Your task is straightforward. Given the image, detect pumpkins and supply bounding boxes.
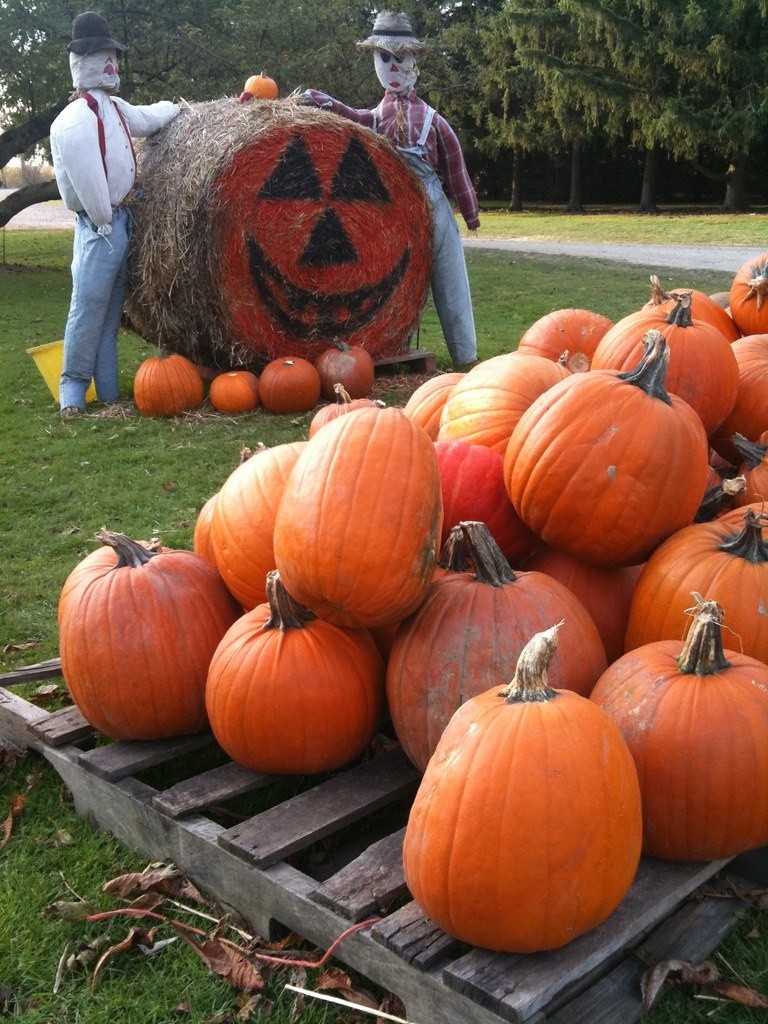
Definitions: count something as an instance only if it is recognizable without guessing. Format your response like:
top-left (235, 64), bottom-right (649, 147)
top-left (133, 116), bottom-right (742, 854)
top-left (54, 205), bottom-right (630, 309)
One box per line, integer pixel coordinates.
top-left (58, 252), bottom-right (768, 951)
top-left (244, 70), bottom-right (278, 99)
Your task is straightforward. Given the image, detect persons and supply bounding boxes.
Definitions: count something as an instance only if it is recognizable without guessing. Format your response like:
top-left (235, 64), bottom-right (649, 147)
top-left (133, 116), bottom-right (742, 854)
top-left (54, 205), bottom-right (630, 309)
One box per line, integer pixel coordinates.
top-left (301, 8), bottom-right (482, 372)
top-left (49, 12), bottom-right (181, 419)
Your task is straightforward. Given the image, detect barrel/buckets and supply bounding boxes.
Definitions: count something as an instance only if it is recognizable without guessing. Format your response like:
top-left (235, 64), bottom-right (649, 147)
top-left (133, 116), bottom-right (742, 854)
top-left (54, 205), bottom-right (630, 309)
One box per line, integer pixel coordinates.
top-left (25, 340), bottom-right (97, 405)
